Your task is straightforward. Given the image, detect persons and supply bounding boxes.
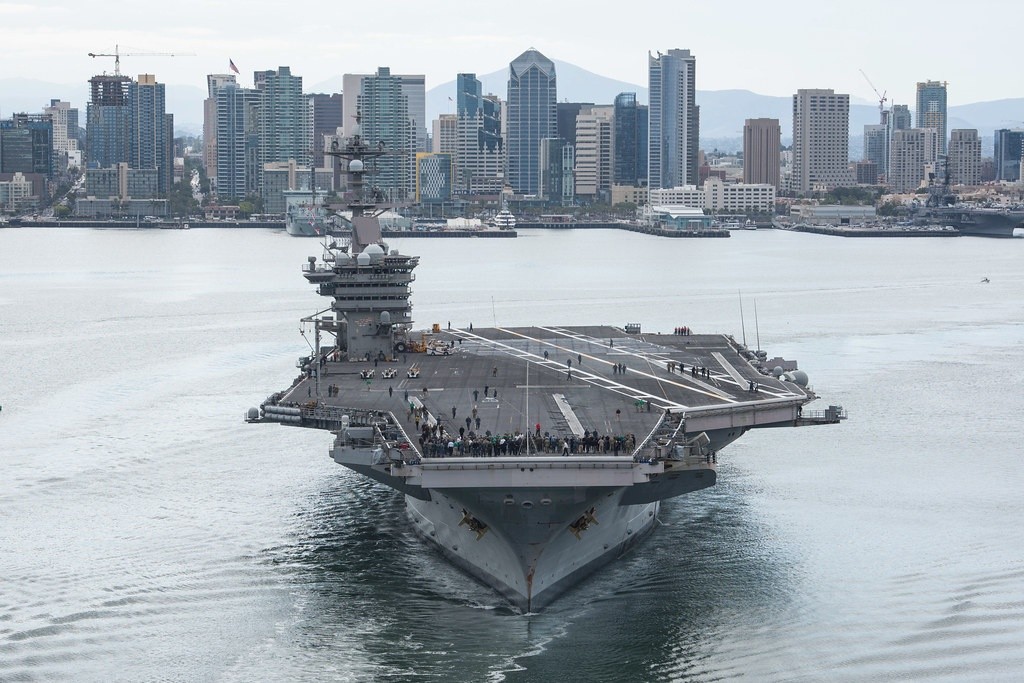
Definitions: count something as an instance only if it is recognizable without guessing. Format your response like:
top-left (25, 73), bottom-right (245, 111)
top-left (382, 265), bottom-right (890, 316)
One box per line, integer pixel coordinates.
top-left (307, 321), bottom-right (650, 457)
top-left (665, 326), bottom-right (761, 392)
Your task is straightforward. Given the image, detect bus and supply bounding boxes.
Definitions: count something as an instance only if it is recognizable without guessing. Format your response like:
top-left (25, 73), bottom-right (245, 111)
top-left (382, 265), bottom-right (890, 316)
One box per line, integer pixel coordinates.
top-left (250, 213), bottom-right (283, 223)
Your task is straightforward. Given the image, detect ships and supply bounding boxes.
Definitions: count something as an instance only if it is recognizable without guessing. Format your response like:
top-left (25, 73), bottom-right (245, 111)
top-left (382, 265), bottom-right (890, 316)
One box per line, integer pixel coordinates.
top-left (242, 133), bottom-right (849, 615)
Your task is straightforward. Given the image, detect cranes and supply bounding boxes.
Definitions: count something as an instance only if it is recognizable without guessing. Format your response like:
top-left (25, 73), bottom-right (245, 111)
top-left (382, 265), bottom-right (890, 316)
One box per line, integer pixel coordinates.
top-left (88, 43), bottom-right (175, 77)
top-left (858, 68), bottom-right (888, 125)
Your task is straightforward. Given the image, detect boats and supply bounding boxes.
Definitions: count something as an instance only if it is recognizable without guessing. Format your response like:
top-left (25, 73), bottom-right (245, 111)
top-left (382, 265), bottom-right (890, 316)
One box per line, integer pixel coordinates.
top-left (282, 186), bottom-right (332, 237)
top-left (493, 197), bottom-right (517, 231)
top-left (708, 215), bottom-right (741, 230)
top-left (743, 217), bottom-right (757, 230)
